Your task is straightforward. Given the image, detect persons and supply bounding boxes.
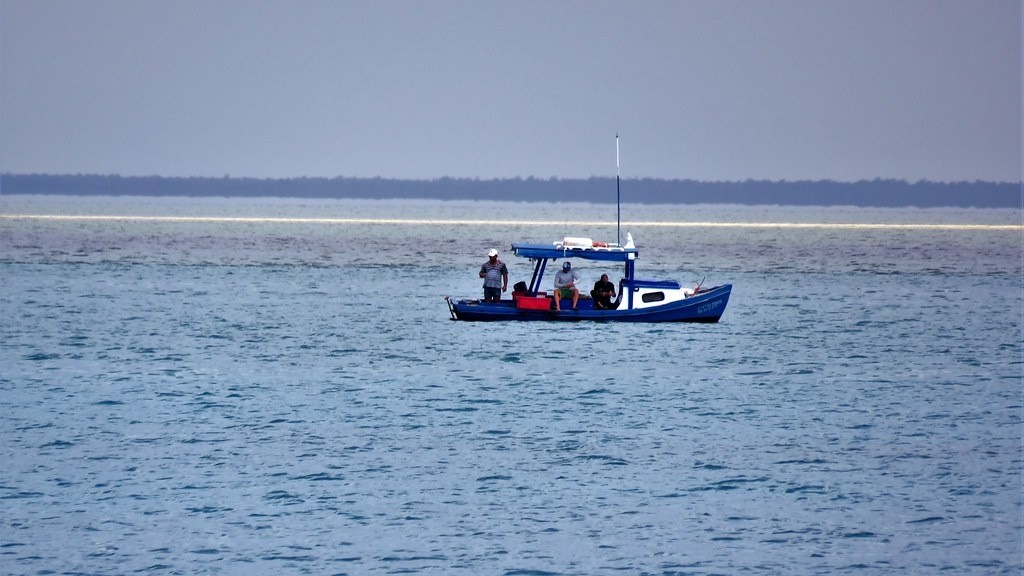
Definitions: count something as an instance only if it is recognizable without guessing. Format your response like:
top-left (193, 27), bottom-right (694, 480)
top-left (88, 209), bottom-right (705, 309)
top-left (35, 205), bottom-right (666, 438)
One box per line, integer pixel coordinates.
top-left (553, 261), bottom-right (582, 311)
top-left (590, 274), bottom-right (616, 310)
top-left (479, 249), bottom-right (508, 304)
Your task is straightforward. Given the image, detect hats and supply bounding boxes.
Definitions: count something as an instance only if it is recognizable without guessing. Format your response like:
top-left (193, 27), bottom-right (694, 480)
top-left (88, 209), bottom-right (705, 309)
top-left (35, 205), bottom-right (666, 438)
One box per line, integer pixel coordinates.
top-left (563, 262), bottom-right (571, 270)
top-left (488, 249), bottom-right (498, 257)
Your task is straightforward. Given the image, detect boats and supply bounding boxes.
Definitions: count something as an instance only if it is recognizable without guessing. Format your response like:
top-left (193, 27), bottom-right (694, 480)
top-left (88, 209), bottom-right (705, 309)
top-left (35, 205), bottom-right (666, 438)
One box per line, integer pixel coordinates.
top-left (445, 132), bottom-right (732, 323)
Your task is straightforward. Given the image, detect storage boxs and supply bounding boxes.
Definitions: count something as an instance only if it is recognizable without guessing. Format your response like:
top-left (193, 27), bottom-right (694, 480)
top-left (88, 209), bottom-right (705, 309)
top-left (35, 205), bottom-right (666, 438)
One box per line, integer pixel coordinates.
top-left (512, 291), bottom-right (547, 300)
top-left (516, 295), bottom-right (551, 310)
